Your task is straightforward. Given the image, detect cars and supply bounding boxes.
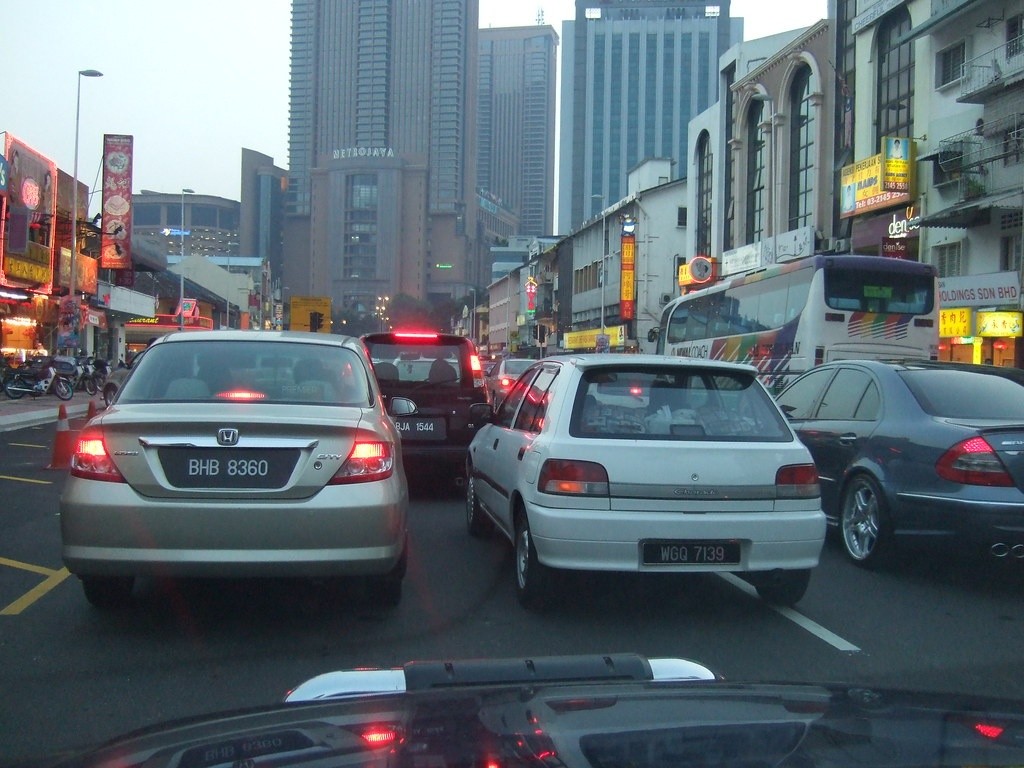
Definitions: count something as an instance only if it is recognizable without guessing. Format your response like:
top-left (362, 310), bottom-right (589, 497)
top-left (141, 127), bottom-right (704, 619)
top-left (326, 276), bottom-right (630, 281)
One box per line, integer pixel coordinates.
top-left (772, 357), bottom-right (1023, 573)
top-left (102, 349), bottom-right (146, 408)
top-left (586, 381), bottom-right (652, 410)
top-left (480, 358), bottom-right (538, 413)
top-left (60, 330), bottom-right (410, 610)
top-left (465, 354), bottom-right (828, 616)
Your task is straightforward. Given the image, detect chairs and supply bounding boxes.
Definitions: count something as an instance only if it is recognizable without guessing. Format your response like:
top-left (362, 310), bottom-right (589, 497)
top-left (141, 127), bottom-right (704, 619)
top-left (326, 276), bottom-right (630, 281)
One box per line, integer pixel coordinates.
top-left (164, 379), bottom-right (213, 400)
top-left (374, 362), bottom-right (399, 387)
top-left (682, 298), bottom-right (925, 341)
top-left (294, 381), bottom-right (338, 403)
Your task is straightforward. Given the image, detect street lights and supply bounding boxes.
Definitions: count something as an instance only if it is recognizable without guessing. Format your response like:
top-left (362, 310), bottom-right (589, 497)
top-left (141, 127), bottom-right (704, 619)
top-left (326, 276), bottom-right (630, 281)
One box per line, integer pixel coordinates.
top-left (469, 289), bottom-right (476, 339)
top-left (225, 242), bottom-right (240, 330)
top-left (259, 269), bottom-right (268, 330)
top-left (751, 94), bottom-right (778, 265)
top-left (374, 296), bottom-right (390, 332)
top-left (179, 188), bottom-right (196, 330)
top-left (68, 69), bottom-right (105, 357)
top-left (590, 195), bottom-right (606, 334)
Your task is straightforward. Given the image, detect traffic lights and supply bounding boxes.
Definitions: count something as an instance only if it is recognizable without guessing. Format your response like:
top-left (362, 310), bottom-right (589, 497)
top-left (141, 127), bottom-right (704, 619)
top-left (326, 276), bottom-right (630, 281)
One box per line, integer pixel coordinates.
top-left (315, 312), bottom-right (324, 329)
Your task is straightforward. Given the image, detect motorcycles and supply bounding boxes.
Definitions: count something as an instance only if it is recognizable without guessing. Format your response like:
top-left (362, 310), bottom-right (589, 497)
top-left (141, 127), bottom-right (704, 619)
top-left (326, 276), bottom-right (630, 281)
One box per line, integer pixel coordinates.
top-left (0, 350), bottom-right (130, 401)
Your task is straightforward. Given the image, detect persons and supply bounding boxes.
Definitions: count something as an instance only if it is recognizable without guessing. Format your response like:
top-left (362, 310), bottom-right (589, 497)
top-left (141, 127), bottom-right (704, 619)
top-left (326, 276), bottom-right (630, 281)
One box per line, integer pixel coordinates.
top-left (36, 343), bottom-right (47, 356)
top-left (858, 176), bottom-right (877, 189)
top-left (844, 186), bottom-right (852, 212)
top-left (646, 380), bottom-right (689, 417)
top-left (199, 362), bottom-right (224, 396)
top-left (293, 359), bottom-right (322, 385)
top-left (890, 140), bottom-right (903, 159)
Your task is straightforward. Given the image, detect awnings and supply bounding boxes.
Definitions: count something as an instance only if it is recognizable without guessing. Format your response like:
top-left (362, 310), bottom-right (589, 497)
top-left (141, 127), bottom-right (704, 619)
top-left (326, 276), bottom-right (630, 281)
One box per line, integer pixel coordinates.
top-left (915, 141), bottom-right (963, 161)
top-left (907, 190), bottom-right (1024, 229)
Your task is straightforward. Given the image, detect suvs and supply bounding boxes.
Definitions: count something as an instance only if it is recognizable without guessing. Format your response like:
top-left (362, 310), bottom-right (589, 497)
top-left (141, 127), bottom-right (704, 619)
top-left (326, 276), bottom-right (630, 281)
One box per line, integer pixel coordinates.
top-left (359, 332), bottom-right (495, 498)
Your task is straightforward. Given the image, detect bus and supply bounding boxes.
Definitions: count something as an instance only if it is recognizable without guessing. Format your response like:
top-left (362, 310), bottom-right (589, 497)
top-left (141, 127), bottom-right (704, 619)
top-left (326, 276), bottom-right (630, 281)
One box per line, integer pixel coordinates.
top-left (647, 254), bottom-right (941, 417)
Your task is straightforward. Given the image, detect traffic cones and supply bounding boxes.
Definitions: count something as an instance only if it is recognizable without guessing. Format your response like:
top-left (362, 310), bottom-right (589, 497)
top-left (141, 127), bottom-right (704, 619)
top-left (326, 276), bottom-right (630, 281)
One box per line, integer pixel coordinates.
top-left (86, 401), bottom-right (98, 422)
top-left (44, 404), bottom-right (74, 470)
